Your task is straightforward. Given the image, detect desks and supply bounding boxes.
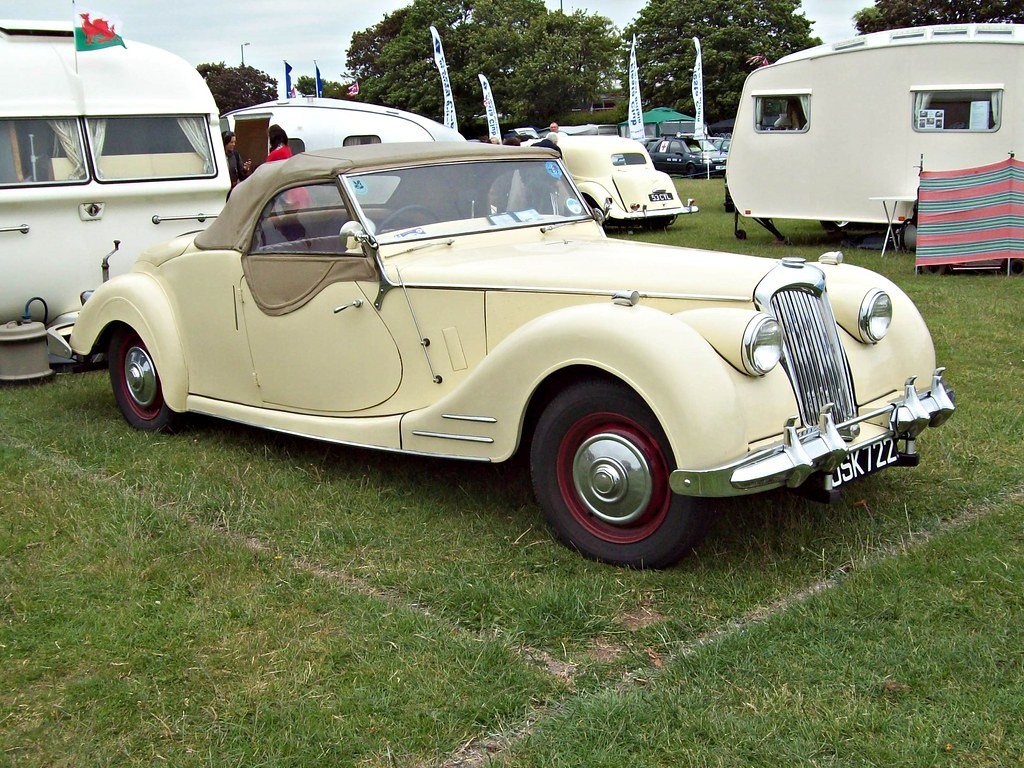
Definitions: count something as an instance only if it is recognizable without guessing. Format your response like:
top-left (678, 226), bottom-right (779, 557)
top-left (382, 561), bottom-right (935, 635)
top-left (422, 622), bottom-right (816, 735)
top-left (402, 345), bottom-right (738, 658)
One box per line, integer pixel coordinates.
top-left (869, 197), bottom-right (918, 257)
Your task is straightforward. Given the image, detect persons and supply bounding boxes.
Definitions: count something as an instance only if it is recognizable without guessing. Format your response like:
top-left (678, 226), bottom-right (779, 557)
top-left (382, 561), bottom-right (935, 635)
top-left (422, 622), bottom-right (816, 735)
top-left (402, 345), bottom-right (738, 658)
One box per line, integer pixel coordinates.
top-left (263, 124), bottom-right (292, 211)
top-left (222, 131), bottom-right (252, 187)
top-left (479, 136), bottom-right (493, 144)
top-left (519, 132), bottom-right (562, 214)
top-left (550, 122), bottom-right (569, 136)
top-left (489, 137), bottom-right (499, 144)
top-left (502, 136), bottom-right (520, 146)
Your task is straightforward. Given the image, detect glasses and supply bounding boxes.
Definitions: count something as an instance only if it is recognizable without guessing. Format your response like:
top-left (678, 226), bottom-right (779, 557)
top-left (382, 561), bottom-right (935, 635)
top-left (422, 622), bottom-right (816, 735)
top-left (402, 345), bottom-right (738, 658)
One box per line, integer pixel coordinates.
top-left (222, 131), bottom-right (233, 141)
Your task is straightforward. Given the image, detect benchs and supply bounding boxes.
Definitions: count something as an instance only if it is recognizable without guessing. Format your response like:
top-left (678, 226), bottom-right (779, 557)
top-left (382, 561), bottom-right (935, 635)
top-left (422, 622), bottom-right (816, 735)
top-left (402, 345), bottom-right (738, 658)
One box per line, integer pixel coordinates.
top-left (258, 235), bottom-right (346, 254)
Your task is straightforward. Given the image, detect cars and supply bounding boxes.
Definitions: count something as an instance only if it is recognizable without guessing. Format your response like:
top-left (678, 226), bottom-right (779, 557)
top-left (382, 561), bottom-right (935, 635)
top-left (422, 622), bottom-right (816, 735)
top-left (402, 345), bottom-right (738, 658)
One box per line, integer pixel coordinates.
top-left (503, 126), bottom-right (540, 143)
top-left (46, 141), bottom-right (956, 567)
top-left (521, 136), bottom-right (698, 227)
top-left (639, 135), bottom-right (731, 178)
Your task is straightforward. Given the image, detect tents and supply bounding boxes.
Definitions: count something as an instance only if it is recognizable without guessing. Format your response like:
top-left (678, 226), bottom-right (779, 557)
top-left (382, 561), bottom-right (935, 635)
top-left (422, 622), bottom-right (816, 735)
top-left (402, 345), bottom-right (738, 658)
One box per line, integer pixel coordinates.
top-left (618, 107), bottom-right (707, 138)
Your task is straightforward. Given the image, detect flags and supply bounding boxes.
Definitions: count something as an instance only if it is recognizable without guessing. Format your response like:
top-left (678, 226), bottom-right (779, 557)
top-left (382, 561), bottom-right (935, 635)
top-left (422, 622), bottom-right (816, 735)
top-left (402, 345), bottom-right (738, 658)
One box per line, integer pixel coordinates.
top-left (72, 2), bottom-right (127, 51)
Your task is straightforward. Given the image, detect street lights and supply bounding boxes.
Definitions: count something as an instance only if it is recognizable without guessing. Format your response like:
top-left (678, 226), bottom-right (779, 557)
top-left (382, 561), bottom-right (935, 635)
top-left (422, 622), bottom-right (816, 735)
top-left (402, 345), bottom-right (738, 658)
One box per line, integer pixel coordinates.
top-left (240, 43), bottom-right (252, 64)
